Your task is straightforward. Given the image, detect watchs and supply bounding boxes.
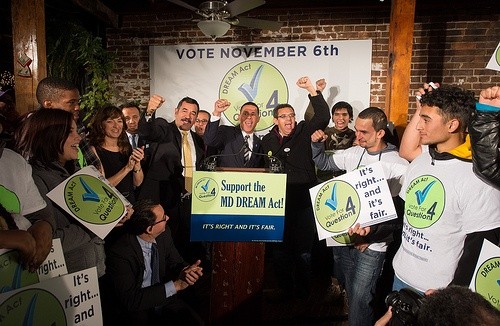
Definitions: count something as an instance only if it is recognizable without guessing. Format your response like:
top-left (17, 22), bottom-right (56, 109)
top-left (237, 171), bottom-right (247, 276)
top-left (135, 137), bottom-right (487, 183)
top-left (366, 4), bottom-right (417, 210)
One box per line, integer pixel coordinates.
top-left (132, 167), bottom-right (142, 174)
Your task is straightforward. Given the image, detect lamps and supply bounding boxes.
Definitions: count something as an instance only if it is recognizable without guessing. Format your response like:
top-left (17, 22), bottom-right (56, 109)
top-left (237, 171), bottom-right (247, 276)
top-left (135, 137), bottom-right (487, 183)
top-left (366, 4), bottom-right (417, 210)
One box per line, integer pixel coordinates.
top-left (197, 17), bottom-right (231, 42)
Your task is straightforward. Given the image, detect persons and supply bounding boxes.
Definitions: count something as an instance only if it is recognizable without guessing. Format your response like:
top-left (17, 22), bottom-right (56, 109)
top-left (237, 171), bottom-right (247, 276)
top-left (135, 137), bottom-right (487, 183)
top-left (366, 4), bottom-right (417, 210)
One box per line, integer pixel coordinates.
top-left (0, 76), bottom-right (500, 326)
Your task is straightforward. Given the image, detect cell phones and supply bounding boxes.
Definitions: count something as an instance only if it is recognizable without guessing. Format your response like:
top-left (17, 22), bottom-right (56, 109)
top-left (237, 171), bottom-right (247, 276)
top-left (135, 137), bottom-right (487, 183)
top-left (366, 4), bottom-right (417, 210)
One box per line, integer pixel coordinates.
top-left (415, 82), bottom-right (438, 101)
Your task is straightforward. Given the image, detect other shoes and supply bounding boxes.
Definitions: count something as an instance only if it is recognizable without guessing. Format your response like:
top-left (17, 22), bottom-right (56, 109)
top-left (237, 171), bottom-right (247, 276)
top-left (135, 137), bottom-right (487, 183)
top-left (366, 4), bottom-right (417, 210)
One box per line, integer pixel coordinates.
top-left (263, 287), bottom-right (287, 299)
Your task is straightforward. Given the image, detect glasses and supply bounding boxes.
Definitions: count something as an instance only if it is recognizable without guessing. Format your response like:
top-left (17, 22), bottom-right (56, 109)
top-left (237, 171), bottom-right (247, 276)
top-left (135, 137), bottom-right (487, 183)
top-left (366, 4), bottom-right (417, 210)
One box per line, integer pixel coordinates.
top-left (152, 215), bottom-right (167, 226)
top-left (194, 119), bottom-right (208, 125)
top-left (277, 114), bottom-right (296, 118)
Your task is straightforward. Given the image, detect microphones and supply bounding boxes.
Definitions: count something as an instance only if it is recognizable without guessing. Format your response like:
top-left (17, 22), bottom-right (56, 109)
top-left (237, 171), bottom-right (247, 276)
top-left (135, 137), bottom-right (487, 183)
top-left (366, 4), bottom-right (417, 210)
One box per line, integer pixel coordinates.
top-left (203, 142), bottom-right (282, 167)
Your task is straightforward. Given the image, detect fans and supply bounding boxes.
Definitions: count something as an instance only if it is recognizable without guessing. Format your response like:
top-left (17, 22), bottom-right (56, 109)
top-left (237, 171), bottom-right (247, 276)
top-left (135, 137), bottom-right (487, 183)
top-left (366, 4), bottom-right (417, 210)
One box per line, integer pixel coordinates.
top-left (167, 0), bottom-right (284, 32)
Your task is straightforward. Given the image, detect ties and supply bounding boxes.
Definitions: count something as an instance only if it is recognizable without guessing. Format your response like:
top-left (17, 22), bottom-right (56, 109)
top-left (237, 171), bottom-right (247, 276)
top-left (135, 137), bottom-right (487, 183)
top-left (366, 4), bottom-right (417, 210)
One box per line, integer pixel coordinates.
top-left (148, 243), bottom-right (160, 286)
top-left (243, 135), bottom-right (251, 166)
top-left (181, 131), bottom-right (193, 194)
top-left (131, 134), bottom-right (137, 149)
